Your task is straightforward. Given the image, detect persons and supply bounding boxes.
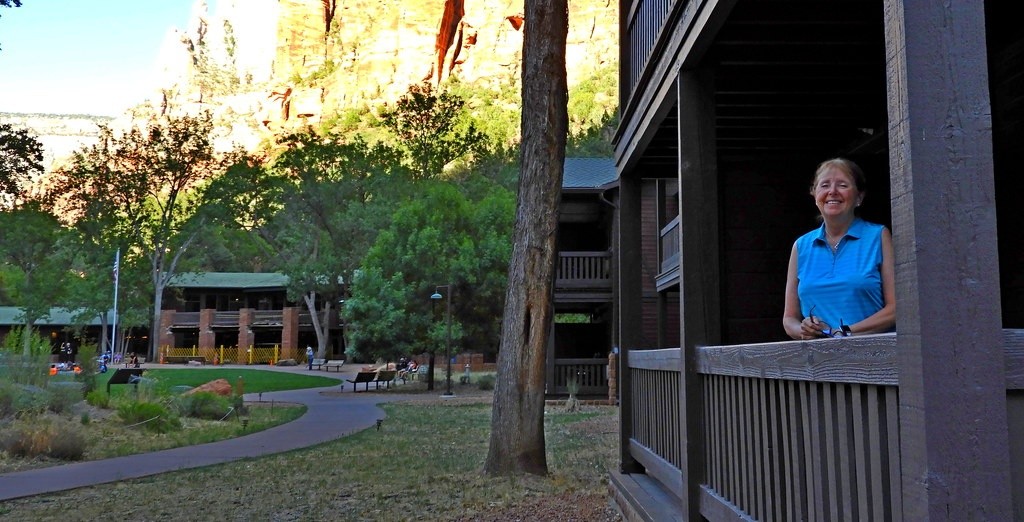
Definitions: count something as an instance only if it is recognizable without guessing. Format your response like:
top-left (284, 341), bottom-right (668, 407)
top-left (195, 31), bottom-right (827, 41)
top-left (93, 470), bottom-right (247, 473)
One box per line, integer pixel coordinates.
top-left (61, 342), bottom-right (140, 377)
top-left (782, 157), bottom-right (896, 341)
top-left (306, 347), bottom-right (313, 370)
top-left (396, 357), bottom-right (417, 379)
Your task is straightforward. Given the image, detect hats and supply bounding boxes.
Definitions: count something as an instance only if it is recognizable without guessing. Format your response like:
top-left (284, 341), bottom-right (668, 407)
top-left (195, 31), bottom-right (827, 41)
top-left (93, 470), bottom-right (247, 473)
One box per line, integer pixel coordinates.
top-left (400, 358), bottom-right (404, 360)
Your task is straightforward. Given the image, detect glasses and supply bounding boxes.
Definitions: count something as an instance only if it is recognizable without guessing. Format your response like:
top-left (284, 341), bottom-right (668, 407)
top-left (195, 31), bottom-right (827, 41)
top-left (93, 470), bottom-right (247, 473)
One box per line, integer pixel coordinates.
top-left (809, 304), bottom-right (846, 337)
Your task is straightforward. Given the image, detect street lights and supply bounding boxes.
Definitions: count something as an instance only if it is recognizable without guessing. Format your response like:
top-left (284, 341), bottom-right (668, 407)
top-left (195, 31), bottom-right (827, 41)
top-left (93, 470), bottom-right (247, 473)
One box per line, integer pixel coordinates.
top-left (431, 285), bottom-right (451, 395)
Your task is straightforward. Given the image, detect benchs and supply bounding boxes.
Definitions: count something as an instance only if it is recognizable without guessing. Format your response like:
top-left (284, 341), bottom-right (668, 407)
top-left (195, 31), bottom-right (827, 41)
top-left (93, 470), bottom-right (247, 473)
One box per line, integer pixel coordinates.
top-left (123, 362), bottom-right (144, 368)
top-left (219, 360), bottom-right (232, 365)
top-left (306, 359), bottom-right (326, 370)
top-left (166, 356), bottom-right (209, 366)
top-left (346, 372), bottom-right (377, 393)
top-left (373, 371), bottom-right (397, 390)
top-left (323, 360), bottom-right (345, 372)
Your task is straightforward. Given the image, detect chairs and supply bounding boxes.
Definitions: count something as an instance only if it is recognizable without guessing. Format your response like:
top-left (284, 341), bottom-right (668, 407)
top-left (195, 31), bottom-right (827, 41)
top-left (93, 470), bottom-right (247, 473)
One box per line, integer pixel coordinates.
top-left (113, 352), bottom-right (123, 365)
